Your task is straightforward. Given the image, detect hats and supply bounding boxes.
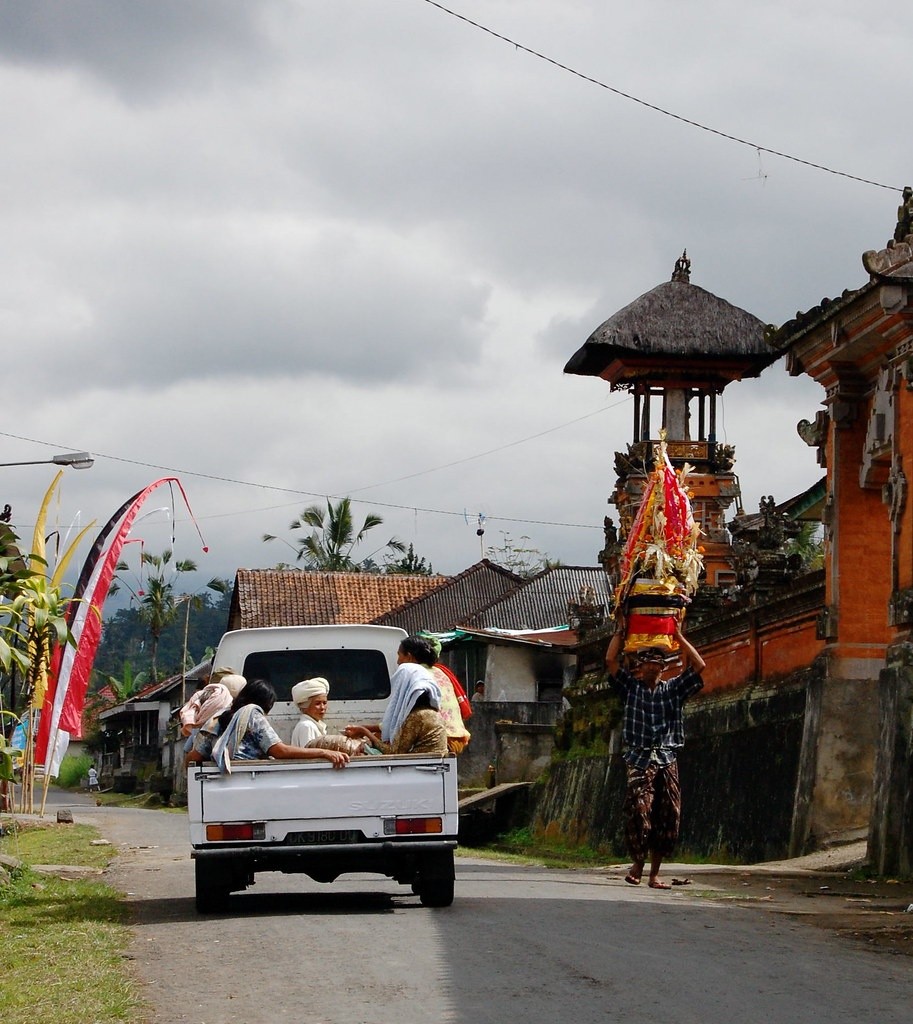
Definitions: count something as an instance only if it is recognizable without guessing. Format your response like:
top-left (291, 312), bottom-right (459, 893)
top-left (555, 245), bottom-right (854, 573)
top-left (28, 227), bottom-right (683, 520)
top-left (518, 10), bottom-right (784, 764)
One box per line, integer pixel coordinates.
top-left (476, 680), bottom-right (484, 686)
top-left (416, 632), bottom-right (442, 657)
top-left (637, 649), bottom-right (664, 667)
top-left (210, 667), bottom-right (240, 683)
top-left (220, 675), bottom-right (247, 698)
top-left (292, 677), bottom-right (330, 710)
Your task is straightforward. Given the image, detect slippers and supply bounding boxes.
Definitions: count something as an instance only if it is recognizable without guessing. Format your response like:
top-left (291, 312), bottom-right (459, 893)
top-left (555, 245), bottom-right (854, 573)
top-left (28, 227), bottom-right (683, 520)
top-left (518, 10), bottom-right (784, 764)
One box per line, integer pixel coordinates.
top-left (625, 870), bottom-right (642, 885)
top-left (648, 879), bottom-right (672, 889)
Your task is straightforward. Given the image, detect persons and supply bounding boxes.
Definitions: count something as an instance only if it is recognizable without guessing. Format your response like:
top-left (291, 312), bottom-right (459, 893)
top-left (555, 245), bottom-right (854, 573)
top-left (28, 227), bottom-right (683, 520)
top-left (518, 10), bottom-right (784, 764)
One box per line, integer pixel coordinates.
top-left (180, 667), bottom-right (247, 767)
top-left (213, 679), bottom-right (350, 775)
top-left (471, 681), bottom-right (485, 700)
top-left (396, 635), bottom-right (471, 754)
top-left (344, 663), bottom-right (448, 754)
top-left (88, 765), bottom-right (101, 793)
top-left (605, 608), bottom-right (706, 891)
top-left (418, 632), bottom-right (472, 720)
top-left (291, 678), bottom-right (329, 747)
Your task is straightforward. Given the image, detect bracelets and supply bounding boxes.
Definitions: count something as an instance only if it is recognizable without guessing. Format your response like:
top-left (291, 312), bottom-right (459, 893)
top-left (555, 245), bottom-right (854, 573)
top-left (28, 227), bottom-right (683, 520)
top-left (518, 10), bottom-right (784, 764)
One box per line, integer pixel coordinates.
top-left (614, 630), bottom-right (623, 636)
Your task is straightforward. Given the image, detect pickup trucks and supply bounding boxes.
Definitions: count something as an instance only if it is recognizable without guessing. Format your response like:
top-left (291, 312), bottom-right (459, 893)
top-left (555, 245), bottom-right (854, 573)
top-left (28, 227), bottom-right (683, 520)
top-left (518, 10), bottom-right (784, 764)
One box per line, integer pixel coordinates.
top-left (188, 623), bottom-right (461, 918)
